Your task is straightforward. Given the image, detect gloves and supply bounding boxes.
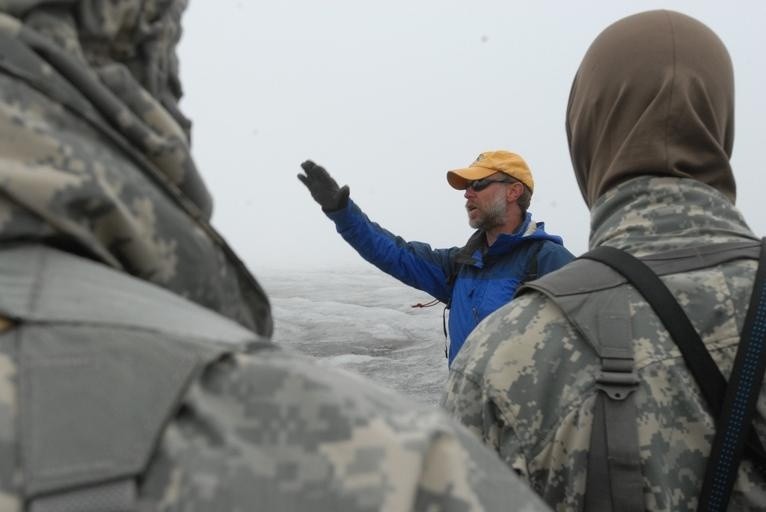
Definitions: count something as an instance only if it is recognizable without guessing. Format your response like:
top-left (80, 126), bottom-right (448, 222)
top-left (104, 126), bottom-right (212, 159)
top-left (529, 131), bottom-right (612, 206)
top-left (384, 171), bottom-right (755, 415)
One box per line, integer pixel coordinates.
top-left (297, 159), bottom-right (350, 212)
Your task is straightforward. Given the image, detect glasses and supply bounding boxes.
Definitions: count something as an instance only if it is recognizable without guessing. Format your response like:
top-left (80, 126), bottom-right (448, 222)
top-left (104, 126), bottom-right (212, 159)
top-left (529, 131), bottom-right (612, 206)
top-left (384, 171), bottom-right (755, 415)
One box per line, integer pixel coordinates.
top-left (464, 178), bottom-right (514, 192)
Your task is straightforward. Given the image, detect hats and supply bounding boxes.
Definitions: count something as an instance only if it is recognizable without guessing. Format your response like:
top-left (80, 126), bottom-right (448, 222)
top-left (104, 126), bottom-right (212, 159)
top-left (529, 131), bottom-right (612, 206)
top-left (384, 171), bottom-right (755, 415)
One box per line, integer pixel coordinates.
top-left (447, 150), bottom-right (535, 192)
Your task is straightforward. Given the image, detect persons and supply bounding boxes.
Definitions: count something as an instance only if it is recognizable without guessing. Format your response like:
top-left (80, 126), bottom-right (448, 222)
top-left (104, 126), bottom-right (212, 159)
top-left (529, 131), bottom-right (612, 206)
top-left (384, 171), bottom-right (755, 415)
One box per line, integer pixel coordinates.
top-left (441, 9), bottom-right (765, 510)
top-left (0, 0), bottom-right (554, 511)
top-left (298, 150), bottom-right (579, 372)
